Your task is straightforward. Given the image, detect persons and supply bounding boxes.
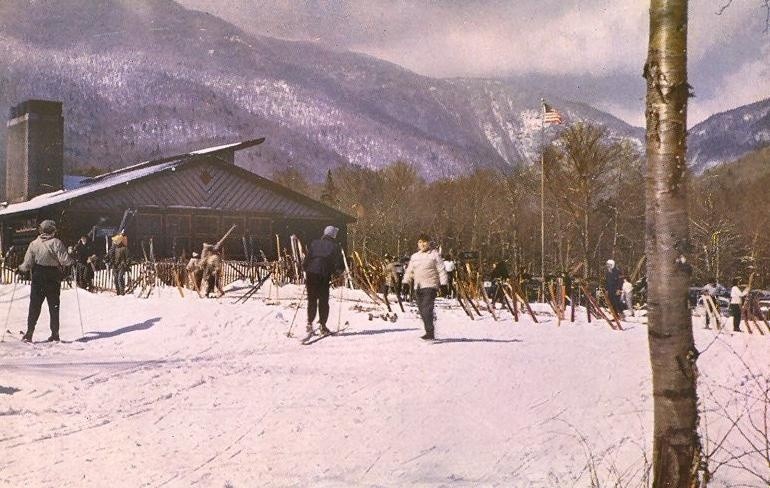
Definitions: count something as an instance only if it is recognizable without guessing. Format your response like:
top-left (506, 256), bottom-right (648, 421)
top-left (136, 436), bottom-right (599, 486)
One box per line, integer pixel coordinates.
top-left (301, 225), bottom-right (344, 334)
top-left (605, 259), bottom-right (626, 321)
top-left (13, 220), bottom-right (76, 343)
top-left (382, 233), bottom-right (456, 340)
top-left (701, 277), bottom-right (726, 330)
top-left (490, 260), bottom-right (509, 309)
top-left (620, 275), bottom-right (635, 317)
top-left (730, 279), bottom-right (750, 331)
top-left (73, 234), bottom-right (96, 291)
top-left (197, 245), bottom-right (225, 298)
top-left (105, 234), bottom-right (131, 296)
top-left (186, 251), bottom-right (199, 290)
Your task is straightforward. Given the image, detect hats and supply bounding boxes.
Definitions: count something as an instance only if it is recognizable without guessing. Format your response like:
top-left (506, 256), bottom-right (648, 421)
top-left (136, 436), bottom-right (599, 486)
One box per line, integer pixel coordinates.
top-left (324, 226), bottom-right (337, 238)
top-left (40, 220), bottom-right (56, 234)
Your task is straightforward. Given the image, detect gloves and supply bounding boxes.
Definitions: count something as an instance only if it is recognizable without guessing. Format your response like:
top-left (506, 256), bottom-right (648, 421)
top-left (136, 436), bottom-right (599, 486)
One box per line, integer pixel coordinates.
top-left (401, 284), bottom-right (410, 295)
top-left (440, 284), bottom-right (449, 297)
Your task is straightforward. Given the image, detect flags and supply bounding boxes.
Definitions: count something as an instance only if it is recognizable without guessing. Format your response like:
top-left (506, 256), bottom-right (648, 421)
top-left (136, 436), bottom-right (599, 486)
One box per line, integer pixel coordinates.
top-left (542, 103), bottom-right (561, 124)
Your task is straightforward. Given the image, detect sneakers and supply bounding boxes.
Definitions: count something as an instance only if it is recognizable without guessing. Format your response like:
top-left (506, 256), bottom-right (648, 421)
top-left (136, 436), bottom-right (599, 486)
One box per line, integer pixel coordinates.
top-left (306, 324), bottom-right (314, 335)
top-left (48, 333), bottom-right (60, 341)
top-left (316, 322), bottom-right (330, 335)
top-left (22, 333), bottom-right (33, 341)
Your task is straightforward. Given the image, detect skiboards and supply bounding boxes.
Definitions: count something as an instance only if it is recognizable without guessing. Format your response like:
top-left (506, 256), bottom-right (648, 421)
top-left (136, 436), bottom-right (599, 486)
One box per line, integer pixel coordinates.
top-left (300, 321), bottom-right (348, 345)
top-left (125, 238), bottom-right (205, 299)
top-left (329, 243), bottom-right (403, 323)
top-left (223, 234), bottom-right (309, 304)
top-left (103, 208), bottom-right (137, 268)
top-left (7, 330), bottom-right (73, 347)
top-left (447, 248), bottom-right (648, 331)
top-left (197, 224), bottom-right (238, 264)
top-left (700, 272), bottom-right (770, 336)
